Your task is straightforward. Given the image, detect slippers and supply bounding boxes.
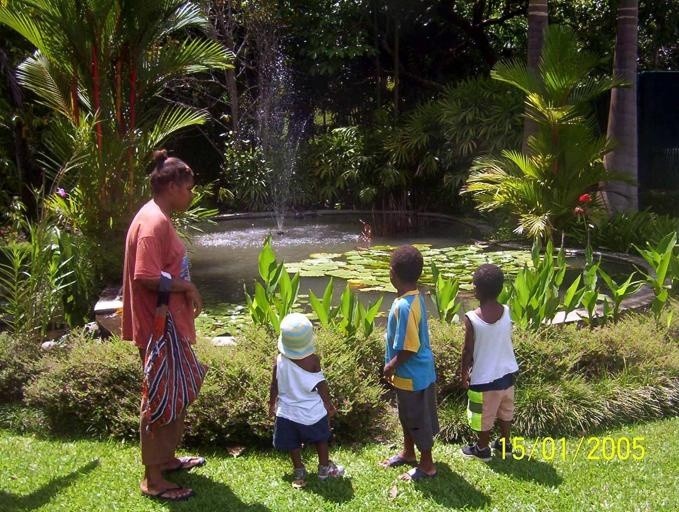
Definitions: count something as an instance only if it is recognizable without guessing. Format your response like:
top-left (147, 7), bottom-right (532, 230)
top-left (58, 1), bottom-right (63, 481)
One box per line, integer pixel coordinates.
top-left (382, 454), bottom-right (416, 467)
top-left (141, 482), bottom-right (195, 501)
top-left (161, 456), bottom-right (205, 472)
top-left (399, 467), bottom-right (437, 482)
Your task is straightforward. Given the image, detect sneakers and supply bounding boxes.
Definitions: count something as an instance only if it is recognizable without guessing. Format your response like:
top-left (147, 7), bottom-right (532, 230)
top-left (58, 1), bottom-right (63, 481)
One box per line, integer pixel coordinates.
top-left (491, 438), bottom-right (512, 456)
top-left (292, 463), bottom-right (308, 488)
top-left (317, 460), bottom-right (344, 481)
top-left (460, 444), bottom-right (492, 462)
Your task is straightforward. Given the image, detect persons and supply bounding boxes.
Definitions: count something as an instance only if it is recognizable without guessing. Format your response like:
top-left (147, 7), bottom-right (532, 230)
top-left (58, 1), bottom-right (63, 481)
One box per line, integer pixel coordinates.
top-left (266, 311), bottom-right (346, 484)
top-left (378, 244), bottom-right (440, 482)
top-left (458, 262), bottom-right (520, 463)
top-left (121, 147), bottom-right (207, 503)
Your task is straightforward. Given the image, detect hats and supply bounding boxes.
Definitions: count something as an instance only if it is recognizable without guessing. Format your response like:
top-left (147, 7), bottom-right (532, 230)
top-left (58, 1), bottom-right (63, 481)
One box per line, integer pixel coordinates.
top-left (278, 313), bottom-right (318, 361)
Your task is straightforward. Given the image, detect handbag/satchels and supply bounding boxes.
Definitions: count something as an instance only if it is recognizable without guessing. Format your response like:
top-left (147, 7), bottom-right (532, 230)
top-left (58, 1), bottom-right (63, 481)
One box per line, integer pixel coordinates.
top-left (145, 311), bottom-right (208, 432)
